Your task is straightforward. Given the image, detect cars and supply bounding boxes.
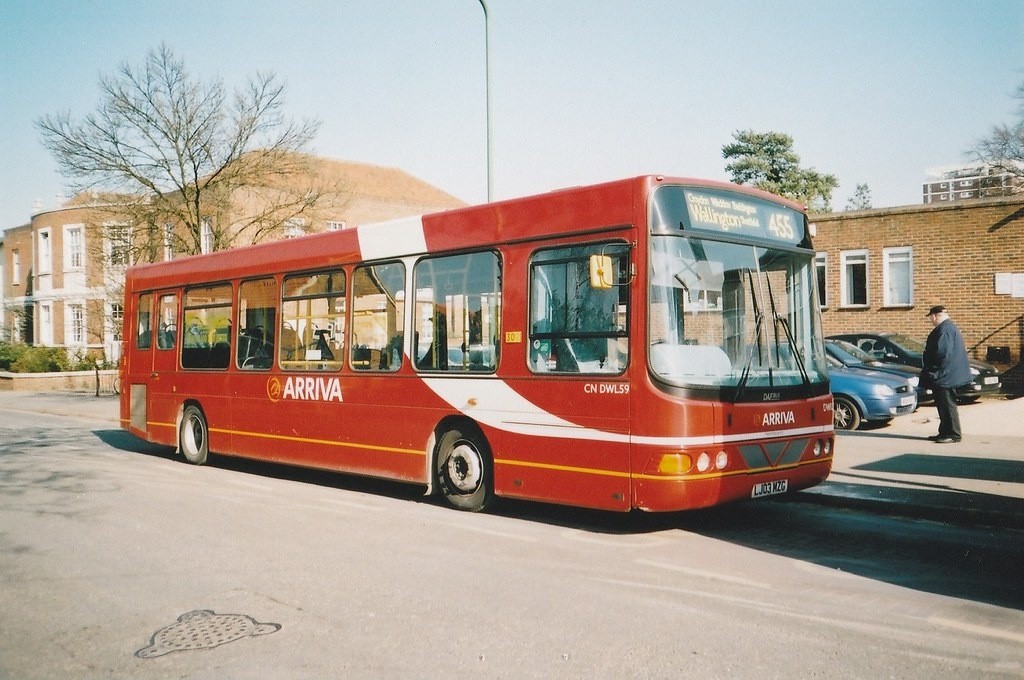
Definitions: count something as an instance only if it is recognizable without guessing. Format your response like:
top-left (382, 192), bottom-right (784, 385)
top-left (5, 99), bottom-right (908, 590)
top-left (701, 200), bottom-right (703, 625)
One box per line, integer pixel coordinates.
top-left (829, 340), bottom-right (934, 403)
top-left (825, 331), bottom-right (1001, 404)
top-left (728, 340), bottom-right (917, 431)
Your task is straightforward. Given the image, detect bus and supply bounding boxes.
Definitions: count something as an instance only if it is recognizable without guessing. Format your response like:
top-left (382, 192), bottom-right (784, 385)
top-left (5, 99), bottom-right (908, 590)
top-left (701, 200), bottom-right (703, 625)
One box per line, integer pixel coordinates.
top-left (119, 174), bottom-right (828, 515)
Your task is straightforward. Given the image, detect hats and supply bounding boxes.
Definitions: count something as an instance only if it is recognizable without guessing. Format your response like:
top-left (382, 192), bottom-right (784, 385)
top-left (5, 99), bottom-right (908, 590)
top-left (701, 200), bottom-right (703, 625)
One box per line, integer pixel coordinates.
top-left (926, 306), bottom-right (948, 317)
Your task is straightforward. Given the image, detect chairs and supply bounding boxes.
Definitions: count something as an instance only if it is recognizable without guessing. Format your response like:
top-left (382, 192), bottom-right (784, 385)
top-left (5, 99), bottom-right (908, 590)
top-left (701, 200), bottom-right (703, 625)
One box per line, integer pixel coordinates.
top-left (860, 342), bottom-right (876, 359)
top-left (555, 298), bottom-right (610, 373)
top-left (138, 316), bottom-right (359, 369)
top-left (874, 341), bottom-right (886, 358)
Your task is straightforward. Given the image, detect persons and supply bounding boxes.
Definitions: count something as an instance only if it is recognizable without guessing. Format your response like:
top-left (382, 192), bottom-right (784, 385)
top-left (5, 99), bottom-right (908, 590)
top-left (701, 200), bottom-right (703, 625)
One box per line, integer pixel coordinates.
top-left (918, 306), bottom-right (973, 443)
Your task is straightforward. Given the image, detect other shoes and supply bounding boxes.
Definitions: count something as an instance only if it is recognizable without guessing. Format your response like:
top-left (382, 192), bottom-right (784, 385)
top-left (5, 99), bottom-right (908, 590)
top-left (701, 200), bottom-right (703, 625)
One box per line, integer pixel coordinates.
top-left (928, 434), bottom-right (941, 440)
top-left (935, 438), bottom-right (961, 443)
